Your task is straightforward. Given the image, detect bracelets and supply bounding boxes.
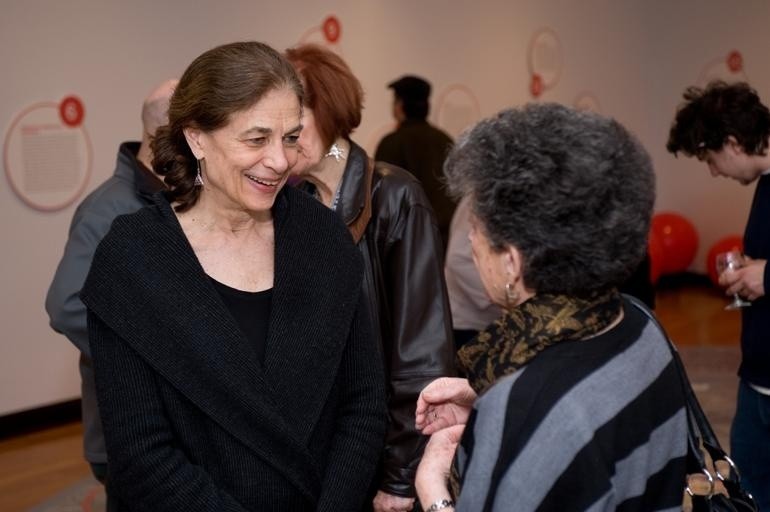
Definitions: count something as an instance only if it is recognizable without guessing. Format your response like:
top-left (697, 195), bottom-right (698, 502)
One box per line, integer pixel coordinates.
top-left (425, 499), bottom-right (455, 512)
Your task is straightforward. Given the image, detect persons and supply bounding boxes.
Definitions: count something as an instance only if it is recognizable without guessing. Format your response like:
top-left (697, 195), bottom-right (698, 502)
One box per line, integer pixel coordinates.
top-left (664, 76), bottom-right (769, 482)
top-left (78, 41), bottom-right (393, 512)
top-left (44, 78), bottom-right (182, 484)
top-left (414, 102), bottom-right (698, 512)
top-left (283, 41), bottom-right (455, 511)
top-left (374, 75), bottom-right (456, 264)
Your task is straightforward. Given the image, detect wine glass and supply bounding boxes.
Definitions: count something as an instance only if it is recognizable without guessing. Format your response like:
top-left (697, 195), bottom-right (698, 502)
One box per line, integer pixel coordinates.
top-left (713, 247), bottom-right (754, 312)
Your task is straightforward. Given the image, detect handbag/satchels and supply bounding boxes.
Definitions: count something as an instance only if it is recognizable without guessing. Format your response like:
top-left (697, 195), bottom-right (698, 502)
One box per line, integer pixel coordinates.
top-left (682, 452), bottom-right (760, 512)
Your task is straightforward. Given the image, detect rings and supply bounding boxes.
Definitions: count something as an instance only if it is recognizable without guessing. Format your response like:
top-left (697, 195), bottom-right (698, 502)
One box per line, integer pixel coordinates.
top-left (430, 411), bottom-right (439, 420)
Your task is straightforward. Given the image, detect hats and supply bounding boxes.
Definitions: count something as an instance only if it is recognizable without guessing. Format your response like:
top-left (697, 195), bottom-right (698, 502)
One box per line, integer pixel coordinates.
top-left (387, 75), bottom-right (432, 101)
